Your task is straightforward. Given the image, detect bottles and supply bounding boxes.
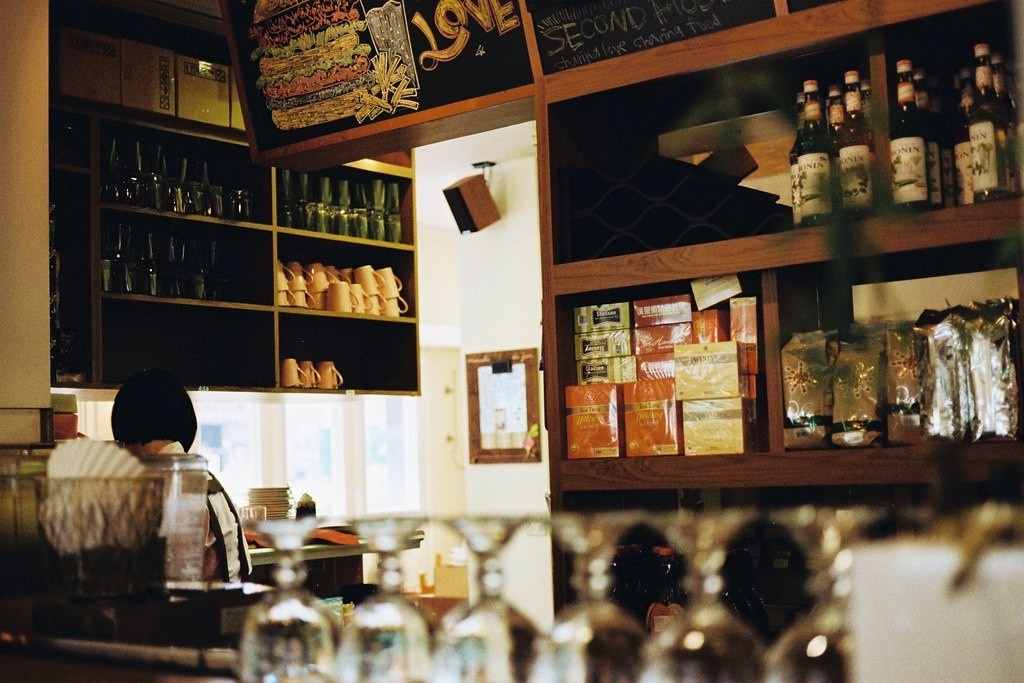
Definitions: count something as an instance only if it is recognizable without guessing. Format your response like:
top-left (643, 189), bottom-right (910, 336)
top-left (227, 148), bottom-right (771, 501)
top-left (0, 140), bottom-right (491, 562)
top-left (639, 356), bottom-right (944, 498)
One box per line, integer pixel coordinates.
top-left (787, 43), bottom-right (1018, 229)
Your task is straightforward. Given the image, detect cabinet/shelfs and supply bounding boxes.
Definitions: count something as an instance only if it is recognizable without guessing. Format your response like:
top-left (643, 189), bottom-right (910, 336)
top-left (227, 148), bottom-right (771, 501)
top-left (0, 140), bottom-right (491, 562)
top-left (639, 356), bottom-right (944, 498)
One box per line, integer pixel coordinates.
top-left (47, 21), bottom-right (423, 399)
top-left (536, 0), bottom-right (1023, 623)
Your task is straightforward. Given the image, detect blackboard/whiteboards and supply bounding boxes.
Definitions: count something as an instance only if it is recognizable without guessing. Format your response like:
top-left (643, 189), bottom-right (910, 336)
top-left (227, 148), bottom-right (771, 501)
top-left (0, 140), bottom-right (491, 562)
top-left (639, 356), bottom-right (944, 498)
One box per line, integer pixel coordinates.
top-left (221, 1), bottom-right (844, 159)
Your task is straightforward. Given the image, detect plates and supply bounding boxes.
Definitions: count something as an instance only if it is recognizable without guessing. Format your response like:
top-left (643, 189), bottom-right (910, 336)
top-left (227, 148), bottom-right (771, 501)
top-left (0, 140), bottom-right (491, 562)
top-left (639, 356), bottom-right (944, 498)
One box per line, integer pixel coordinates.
top-left (247, 487), bottom-right (292, 520)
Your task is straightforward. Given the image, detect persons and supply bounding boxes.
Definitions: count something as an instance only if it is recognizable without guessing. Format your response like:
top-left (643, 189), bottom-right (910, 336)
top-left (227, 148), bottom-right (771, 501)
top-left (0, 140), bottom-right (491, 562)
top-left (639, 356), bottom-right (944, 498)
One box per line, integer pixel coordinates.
top-left (111, 368), bottom-right (252, 586)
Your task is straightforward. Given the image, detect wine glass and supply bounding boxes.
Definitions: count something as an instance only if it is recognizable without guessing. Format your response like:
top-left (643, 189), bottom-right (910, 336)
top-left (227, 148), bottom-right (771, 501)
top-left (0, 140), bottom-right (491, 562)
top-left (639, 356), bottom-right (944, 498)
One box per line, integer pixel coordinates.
top-left (234, 503), bottom-right (1024, 683)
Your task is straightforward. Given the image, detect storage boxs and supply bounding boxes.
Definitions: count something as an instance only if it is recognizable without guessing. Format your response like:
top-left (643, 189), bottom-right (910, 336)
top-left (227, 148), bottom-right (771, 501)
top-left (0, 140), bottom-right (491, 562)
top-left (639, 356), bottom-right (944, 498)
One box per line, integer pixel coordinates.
top-left (120, 40), bottom-right (175, 116)
top-left (57, 30), bottom-right (121, 104)
top-left (230, 68), bottom-right (244, 130)
top-left (564, 273), bottom-right (759, 458)
top-left (174, 50), bottom-right (230, 128)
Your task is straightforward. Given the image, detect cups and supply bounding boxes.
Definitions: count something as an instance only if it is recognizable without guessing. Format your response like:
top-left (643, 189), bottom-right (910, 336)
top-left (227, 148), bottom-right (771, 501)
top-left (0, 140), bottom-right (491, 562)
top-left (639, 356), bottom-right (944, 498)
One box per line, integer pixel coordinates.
top-left (99, 137), bottom-right (252, 222)
top-left (280, 357), bottom-right (344, 390)
top-left (100, 214), bottom-right (234, 300)
top-left (277, 170), bottom-right (402, 244)
top-left (277, 259), bottom-right (408, 317)
